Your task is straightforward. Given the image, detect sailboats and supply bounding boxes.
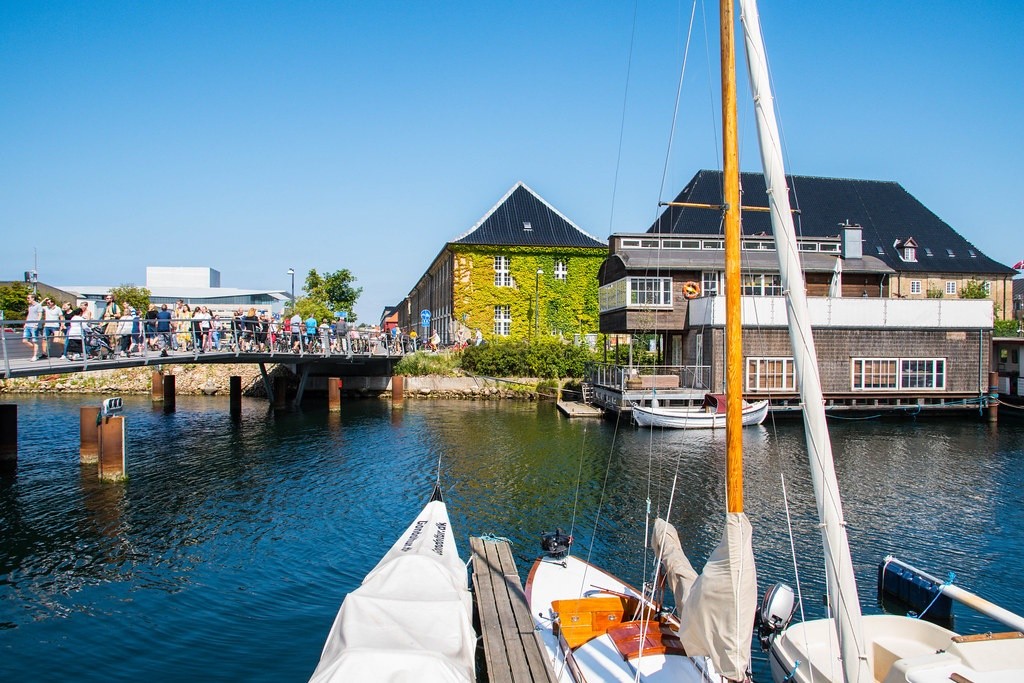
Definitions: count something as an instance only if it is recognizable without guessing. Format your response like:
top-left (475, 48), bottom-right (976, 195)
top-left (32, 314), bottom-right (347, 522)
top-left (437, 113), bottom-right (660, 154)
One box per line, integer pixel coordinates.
top-left (523, 1), bottom-right (877, 683)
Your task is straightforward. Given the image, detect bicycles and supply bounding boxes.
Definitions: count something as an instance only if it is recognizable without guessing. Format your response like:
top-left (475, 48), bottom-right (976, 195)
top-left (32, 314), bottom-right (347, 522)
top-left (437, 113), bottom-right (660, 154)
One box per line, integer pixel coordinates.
top-left (419, 342), bottom-right (446, 353)
top-left (351, 342), bottom-right (369, 354)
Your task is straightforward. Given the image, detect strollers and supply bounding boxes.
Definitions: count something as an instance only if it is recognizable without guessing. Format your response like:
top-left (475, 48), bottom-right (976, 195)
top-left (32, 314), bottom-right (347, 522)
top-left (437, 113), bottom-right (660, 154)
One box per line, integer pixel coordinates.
top-left (85, 329), bottom-right (118, 360)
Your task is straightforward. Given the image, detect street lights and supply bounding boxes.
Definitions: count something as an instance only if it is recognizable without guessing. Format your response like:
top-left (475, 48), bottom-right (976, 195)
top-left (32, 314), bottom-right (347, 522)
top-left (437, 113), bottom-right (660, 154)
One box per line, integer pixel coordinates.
top-left (286, 268), bottom-right (295, 315)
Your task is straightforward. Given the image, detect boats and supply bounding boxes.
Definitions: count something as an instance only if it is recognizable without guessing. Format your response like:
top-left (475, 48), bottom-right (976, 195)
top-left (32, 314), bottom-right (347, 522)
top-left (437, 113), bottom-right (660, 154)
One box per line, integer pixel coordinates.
top-left (759, 581), bottom-right (1024, 683)
top-left (309, 449), bottom-right (475, 682)
top-left (628, 396), bottom-right (770, 428)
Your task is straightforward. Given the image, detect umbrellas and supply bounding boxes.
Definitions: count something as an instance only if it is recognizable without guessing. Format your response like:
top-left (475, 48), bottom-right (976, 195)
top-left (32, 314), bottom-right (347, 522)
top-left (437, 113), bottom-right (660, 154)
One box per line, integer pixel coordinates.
top-left (829, 255), bottom-right (842, 296)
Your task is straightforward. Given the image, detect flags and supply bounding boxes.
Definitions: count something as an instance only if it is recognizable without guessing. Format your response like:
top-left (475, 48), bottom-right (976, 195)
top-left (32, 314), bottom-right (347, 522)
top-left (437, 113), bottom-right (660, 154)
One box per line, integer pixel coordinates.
top-left (1012, 259), bottom-right (1024, 269)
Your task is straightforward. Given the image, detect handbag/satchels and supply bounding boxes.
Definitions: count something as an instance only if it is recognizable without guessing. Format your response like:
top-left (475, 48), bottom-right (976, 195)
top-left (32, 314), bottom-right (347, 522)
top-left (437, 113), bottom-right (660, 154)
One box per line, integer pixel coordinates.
top-left (53, 328), bottom-right (65, 345)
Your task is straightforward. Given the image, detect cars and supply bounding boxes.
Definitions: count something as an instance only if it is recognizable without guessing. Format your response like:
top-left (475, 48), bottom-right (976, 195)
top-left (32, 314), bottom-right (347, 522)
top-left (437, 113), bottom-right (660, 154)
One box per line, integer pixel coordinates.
top-left (381, 333), bottom-right (421, 352)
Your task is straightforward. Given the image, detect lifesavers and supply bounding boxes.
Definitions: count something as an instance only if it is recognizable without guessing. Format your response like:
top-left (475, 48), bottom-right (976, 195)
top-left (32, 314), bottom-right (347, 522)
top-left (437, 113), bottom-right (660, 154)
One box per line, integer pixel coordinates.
top-left (683, 281), bottom-right (699, 299)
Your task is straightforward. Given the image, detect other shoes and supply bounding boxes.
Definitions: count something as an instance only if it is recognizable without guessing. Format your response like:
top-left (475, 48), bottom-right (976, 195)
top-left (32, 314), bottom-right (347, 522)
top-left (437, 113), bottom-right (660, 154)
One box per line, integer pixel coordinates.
top-left (60, 355), bottom-right (66, 360)
top-left (30, 356), bottom-right (40, 362)
top-left (120, 350), bottom-right (127, 356)
top-left (40, 354), bottom-right (48, 359)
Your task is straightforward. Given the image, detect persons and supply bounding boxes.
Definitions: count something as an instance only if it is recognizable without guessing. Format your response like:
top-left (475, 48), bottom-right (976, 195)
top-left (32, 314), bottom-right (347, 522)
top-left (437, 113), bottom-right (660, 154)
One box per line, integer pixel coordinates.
top-left (23, 292), bottom-right (421, 362)
top-left (474, 328), bottom-right (482, 346)
top-left (429, 329), bottom-right (440, 349)
